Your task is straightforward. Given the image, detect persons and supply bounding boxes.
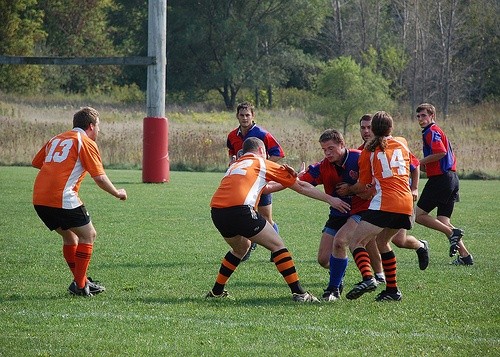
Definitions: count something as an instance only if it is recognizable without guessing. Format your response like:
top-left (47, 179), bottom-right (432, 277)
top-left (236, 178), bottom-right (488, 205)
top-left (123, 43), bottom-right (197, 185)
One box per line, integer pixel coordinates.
top-left (226, 103), bottom-right (285, 262)
top-left (415, 103), bottom-right (474, 265)
top-left (31, 107), bottom-right (128, 298)
top-left (283, 111), bottom-right (429, 302)
top-left (205, 136), bottom-right (350, 303)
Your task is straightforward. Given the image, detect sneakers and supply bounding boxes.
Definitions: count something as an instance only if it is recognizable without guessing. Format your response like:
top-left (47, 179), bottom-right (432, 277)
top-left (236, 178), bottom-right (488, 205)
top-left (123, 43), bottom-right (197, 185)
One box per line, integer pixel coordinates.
top-left (415, 238), bottom-right (430, 270)
top-left (450, 255), bottom-right (475, 266)
top-left (447, 228), bottom-right (463, 257)
top-left (374, 271), bottom-right (386, 284)
top-left (292, 290), bottom-right (321, 303)
top-left (374, 290), bottom-right (402, 301)
top-left (203, 290), bottom-right (236, 300)
top-left (322, 283), bottom-right (344, 299)
top-left (345, 277), bottom-right (378, 299)
top-left (69, 277), bottom-right (106, 298)
